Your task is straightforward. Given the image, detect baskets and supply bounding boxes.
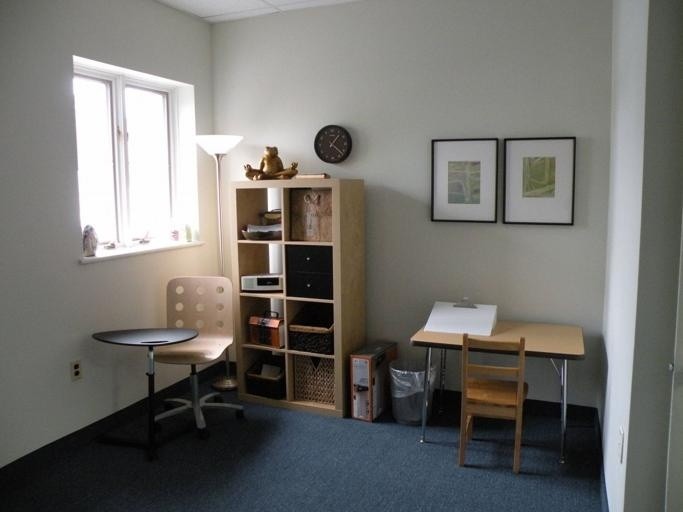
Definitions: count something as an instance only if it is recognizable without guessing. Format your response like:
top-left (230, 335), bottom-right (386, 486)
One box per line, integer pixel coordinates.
top-left (294, 355), bottom-right (336, 405)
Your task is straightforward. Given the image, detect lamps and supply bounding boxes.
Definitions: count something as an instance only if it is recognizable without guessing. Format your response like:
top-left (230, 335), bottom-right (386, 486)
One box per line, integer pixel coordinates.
top-left (193, 130), bottom-right (245, 390)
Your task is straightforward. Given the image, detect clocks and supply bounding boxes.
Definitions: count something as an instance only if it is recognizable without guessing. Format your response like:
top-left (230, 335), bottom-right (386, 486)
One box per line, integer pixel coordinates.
top-left (312, 122), bottom-right (352, 167)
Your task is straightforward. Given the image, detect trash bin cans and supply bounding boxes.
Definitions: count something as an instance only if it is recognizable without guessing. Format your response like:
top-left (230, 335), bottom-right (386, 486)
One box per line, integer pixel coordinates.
top-left (388, 358), bottom-right (438, 426)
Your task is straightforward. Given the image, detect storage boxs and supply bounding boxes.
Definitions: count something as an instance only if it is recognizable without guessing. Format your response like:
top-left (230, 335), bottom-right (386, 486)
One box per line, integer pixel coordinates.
top-left (245, 364), bottom-right (285, 402)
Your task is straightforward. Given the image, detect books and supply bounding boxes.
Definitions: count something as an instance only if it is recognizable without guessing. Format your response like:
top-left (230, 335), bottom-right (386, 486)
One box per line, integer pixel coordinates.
top-left (295, 173), bottom-right (329, 178)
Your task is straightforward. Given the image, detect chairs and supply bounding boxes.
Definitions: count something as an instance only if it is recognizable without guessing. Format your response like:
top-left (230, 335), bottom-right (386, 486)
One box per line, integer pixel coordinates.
top-left (146, 275), bottom-right (243, 443)
top-left (457, 332), bottom-right (533, 475)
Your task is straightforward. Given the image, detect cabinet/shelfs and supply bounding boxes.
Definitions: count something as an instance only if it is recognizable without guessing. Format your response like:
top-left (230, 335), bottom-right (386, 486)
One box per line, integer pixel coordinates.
top-left (234, 176), bottom-right (368, 423)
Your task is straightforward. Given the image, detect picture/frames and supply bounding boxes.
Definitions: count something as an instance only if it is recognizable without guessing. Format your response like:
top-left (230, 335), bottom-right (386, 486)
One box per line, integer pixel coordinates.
top-left (502, 134), bottom-right (577, 227)
top-left (429, 136), bottom-right (499, 225)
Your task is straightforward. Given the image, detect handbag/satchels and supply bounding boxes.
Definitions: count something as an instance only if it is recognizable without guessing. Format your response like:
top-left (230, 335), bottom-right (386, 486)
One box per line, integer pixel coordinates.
top-left (248, 311), bottom-right (285, 349)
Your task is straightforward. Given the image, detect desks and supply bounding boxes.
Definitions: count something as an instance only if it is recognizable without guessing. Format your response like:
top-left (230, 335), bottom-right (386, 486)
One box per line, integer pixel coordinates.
top-left (89, 325), bottom-right (197, 459)
top-left (408, 313), bottom-right (585, 465)
